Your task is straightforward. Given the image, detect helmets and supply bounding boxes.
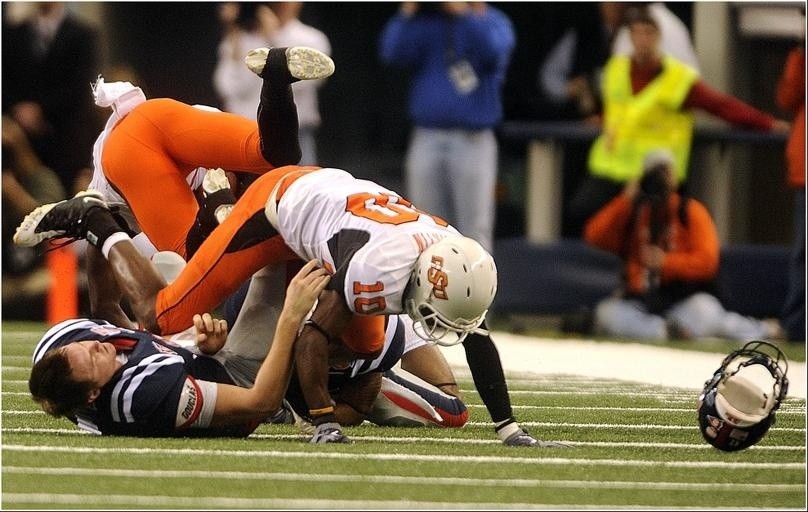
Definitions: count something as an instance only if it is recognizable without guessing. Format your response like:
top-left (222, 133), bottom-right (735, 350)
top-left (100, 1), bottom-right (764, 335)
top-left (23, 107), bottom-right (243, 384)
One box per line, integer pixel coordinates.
top-left (405, 236), bottom-right (497, 346)
top-left (696, 340), bottom-right (787, 451)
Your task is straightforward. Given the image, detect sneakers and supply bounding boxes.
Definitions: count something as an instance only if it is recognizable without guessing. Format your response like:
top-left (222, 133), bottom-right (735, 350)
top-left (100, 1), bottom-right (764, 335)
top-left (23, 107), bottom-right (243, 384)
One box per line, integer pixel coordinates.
top-left (199, 168), bottom-right (236, 223)
top-left (13, 189), bottom-right (108, 248)
top-left (244, 46), bottom-right (335, 79)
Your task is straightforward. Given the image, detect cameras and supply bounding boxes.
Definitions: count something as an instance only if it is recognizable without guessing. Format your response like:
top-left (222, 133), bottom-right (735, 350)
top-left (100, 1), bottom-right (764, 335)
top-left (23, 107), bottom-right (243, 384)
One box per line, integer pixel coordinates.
top-left (235, 2), bottom-right (261, 31)
top-left (638, 170), bottom-right (667, 198)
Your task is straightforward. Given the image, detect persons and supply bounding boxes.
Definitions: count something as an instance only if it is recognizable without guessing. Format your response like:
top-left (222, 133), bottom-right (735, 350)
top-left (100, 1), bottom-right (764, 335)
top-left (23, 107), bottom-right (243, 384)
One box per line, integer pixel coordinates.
top-left (2, 1), bottom-right (806, 449)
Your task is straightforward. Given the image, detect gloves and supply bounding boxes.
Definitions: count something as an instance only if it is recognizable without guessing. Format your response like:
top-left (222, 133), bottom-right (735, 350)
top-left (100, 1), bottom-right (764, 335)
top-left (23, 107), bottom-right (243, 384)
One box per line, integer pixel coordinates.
top-left (494, 418), bottom-right (569, 449)
top-left (308, 422), bottom-right (355, 445)
